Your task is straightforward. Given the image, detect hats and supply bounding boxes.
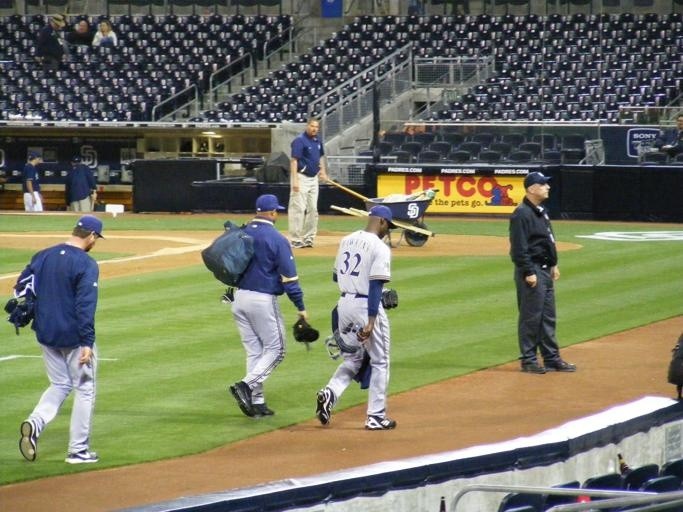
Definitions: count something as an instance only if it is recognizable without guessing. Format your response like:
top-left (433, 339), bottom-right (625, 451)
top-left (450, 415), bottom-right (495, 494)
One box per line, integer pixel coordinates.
top-left (26, 152), bottom-right (44, 160)
top-left (368, 204), bottom-right (397, 230)
top-left (254, 194), bottom-right (286, 214)
top-left (70, 153), bottom-right (83, 164)
top-left (523, 172), bottom-right (552, 189)
top-left (76, 216), bottom-right (106, 238)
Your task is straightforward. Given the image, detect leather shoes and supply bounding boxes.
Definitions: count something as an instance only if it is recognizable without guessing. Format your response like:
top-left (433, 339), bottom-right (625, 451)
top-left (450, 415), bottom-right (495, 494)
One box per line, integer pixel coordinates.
top-left (364, 412), bottom-right (394, 431)
top-left (315, 388), bottom-right (335, 425)
top-left (521, 360), bottom-right (545, 377)
top-left (64, 451), bottom-right (100, 468)
top-left (19, 417), bottom-right (41, 463)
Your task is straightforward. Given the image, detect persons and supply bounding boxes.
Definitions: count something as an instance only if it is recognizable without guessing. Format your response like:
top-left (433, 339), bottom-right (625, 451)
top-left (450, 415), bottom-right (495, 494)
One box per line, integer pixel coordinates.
top-left (287, 119), bottom-right (327, 249)
top-left (63, 155), bottom-right (98, 211)
top-left (315, 205), bottom-right (397, 432)
top-left (92, 19), bottom-right (118, 47)
top-left (13, 216), bottom-right (106, 463)
top-left (508, 170), bottom-right (576, 373)
top-left (21, 151), bottom-right (44, 212)
top-left (228, 194), bottom-right (309, 416)
top-left (66, 19), bottom-right (90, 43)
top-left (35, 14), bottom-right (66, 57)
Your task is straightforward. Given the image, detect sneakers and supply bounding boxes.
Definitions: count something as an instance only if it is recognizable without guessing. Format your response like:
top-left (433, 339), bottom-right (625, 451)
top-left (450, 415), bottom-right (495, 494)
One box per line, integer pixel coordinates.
top-left (545, 358), bottom-right (575, 375)
top-left (228, 379), bottom-right (274, 422)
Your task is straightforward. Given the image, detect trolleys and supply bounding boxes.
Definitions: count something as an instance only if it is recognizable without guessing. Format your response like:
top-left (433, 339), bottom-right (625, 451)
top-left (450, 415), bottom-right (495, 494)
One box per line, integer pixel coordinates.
top-left (323, 174), bottom-right (437, 247)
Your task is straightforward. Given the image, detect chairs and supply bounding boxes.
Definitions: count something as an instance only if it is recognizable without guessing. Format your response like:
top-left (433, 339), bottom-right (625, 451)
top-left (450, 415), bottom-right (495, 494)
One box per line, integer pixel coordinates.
top-left (497, 457), bottom-right (683, 512)
top-left (0, 0), bottom-right (683, 185)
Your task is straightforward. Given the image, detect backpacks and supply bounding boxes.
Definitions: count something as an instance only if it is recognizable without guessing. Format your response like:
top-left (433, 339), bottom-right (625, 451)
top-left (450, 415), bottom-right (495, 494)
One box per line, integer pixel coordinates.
top-left (199, 220), bottom-right (255, 288)
